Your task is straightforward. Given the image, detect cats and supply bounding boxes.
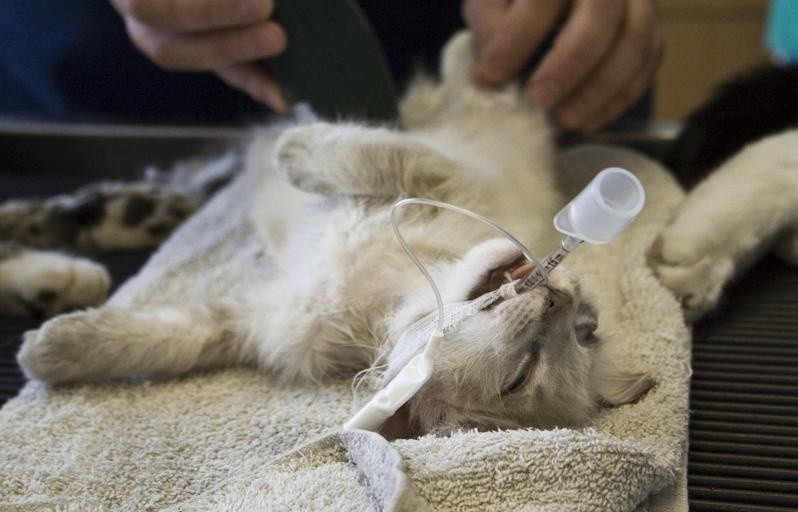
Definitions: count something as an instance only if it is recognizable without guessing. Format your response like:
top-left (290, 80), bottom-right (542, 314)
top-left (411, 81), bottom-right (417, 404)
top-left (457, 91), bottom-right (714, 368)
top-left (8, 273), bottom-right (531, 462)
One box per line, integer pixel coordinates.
top-left (0, 29), bottom-right (657, 435)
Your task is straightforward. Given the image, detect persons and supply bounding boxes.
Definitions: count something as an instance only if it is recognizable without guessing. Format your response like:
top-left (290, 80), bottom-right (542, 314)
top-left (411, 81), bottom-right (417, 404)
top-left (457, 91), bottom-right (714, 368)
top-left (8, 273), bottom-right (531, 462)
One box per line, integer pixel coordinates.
top-left (113, 0), bottom-right (663, 134)
